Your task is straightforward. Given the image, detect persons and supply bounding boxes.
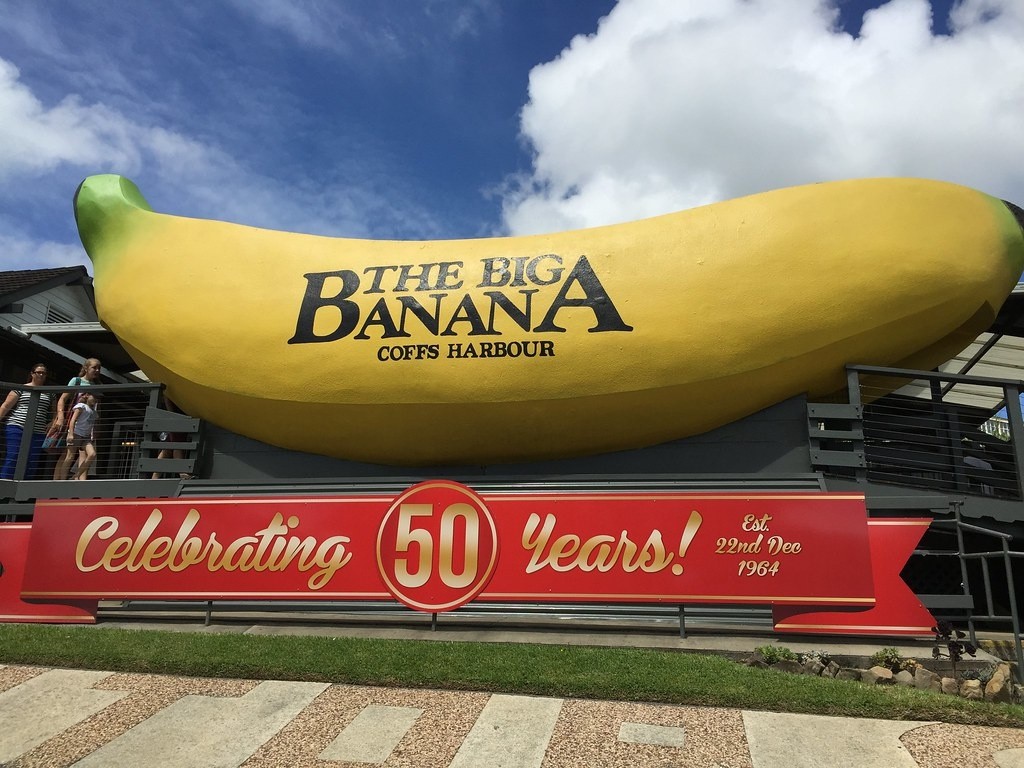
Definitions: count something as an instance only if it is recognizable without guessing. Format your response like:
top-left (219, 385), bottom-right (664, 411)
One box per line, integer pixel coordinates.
top-left (0, 357), bottom-right (102, 480)
top-left (152, 388), bottom-right (191, 479)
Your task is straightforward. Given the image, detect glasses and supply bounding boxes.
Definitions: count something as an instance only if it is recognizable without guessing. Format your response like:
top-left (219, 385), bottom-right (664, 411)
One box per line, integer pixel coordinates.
top-left (33, 371), bottom-right (47, 376)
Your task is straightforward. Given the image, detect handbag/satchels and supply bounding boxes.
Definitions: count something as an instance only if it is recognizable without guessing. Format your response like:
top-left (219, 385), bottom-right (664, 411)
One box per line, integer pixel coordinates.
top-left (41, 419), bottom-right (69, 453)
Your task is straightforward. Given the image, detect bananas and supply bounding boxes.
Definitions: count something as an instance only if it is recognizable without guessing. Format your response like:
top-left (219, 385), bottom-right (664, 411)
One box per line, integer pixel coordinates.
top-left (74, 174), bottom-right (1024, 459)
